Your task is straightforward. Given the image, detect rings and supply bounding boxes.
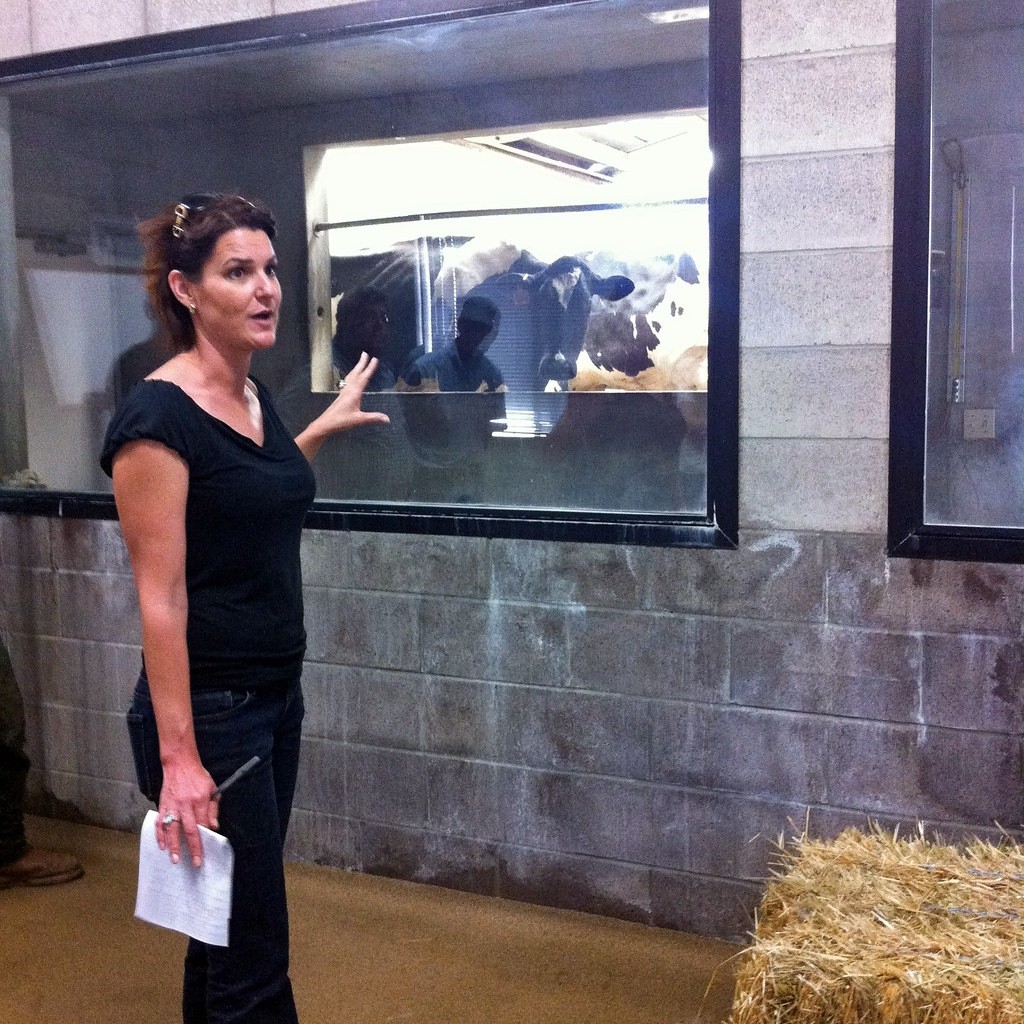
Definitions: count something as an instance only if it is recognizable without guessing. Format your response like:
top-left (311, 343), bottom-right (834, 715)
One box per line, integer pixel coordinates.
top-left (160, 813), bottom-right (180, 828)
top-left (336, 379), bottom-right (346, 389)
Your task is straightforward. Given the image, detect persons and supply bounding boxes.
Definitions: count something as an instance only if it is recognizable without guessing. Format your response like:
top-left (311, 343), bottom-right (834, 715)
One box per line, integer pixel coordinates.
top-left (0, 637), bottom-right (83, 890)
top-left (100, 189), bottom-right (389, 1024)
top-left (329, 283), bottom-right (504, 396)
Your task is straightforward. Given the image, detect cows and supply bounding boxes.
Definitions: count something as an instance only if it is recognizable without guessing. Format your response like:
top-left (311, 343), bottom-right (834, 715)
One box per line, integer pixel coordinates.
top-left (329, 227), bottom-right (711, 519)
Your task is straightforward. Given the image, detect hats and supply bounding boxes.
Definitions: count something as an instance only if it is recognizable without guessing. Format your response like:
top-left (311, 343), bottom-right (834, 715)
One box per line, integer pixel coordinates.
top-left (461, 297), bottom-right (502, 328)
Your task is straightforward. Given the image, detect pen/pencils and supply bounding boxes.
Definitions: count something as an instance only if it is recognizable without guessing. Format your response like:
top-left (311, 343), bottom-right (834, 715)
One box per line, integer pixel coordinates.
top-left (211, 756), bottom-right (260, 801)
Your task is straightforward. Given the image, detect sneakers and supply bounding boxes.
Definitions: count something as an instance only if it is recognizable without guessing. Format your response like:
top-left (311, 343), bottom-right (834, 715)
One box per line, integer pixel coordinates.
top-left (0, 843), bottom-right (83, 890)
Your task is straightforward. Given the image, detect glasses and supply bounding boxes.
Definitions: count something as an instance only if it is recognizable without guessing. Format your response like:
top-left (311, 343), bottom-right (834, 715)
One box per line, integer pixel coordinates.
top-left (169, 191), bottom-right (260, 270)
